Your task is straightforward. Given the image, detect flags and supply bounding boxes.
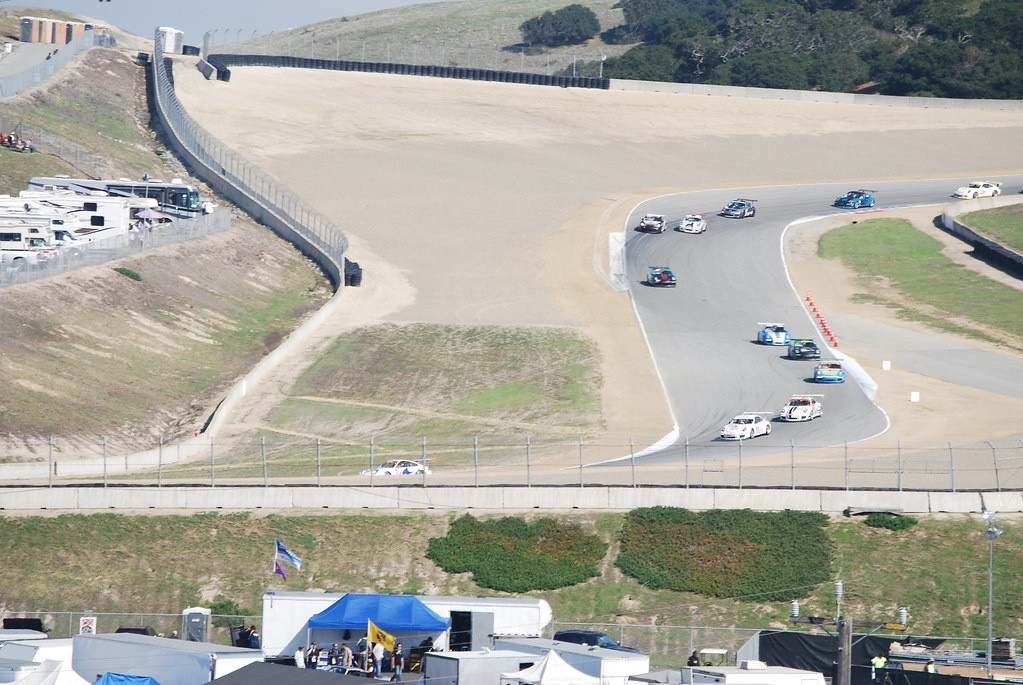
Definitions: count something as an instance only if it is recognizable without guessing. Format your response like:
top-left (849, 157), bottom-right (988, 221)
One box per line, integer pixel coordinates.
top-left (275, 561), bottom-right (288, 581)
top-left (369, 620), bottom-right (396, 652)
top-left (277, 540), bottom-right (304, 571)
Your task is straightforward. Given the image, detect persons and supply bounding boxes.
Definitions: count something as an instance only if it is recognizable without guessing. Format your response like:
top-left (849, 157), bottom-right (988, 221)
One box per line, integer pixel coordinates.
top-left (294, 645), bottom-right (305, 669)
top-left (419, 637), bottom-right (433, 647)
top-left (342, 643), bottom-right (352, 667)
top-left (390, 643), bottom-right (404, 681)
top-left (356, 633), bottom-right (372, 670)
top-left (329, 644), bottom-right (339, 664)
top-left (307, 641), bottom-right (319, 669)
top-left (246, 625), bottom-right (257, 640)
top-left (372, 643), bottom-right (384, 678)
top-left (687, 651), bottom-right (700, 666)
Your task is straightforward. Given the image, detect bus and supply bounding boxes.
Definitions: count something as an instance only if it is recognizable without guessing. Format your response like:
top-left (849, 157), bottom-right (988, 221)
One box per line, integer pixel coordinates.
top-left (28, 173), bottom-right (204, 226)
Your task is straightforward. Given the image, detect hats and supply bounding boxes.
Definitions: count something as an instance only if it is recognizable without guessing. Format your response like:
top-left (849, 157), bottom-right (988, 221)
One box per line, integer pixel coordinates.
top-left (311, 642), bottom-right (317, 645)
top-left (172, 630), bottom-right (178, 635)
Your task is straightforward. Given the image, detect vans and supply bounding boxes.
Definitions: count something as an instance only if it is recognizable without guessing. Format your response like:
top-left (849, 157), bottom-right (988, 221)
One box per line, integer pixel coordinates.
top-left (0, 191), bottom-right (158, 269)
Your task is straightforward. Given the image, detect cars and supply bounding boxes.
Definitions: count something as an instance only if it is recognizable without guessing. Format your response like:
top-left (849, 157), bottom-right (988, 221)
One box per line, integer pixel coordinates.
top-left (953, 181), bottom-right (1003, 200)
top-left (720, 198), bottom-right (758, 218)
top-left (553, 629), bottom-right (640, 654)
top-left (720, 412), bottom-right (772, 440)
top-left (639, 213), bottom-right (667, 234)
top-left (834, 189), bottom-right (878, 209)
top-left (647, 266), bottom-right (677, 287)
top-left (359, 458), bottom-right (432, 478)
top-left (813, 358), bottom-right (845, 384)
top-left (757, 322), bottom-right (791, 346)
top-left (780, 393), bottom-right (823, 421)
top-left (678, 214), bottom-right (707, 234)
top-left (788, 338), bottom-right (821, 361)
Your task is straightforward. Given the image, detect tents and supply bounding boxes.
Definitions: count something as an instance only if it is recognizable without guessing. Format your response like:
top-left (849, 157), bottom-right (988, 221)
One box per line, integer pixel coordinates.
top-left (307, 593), bottom-right (452, 651)
